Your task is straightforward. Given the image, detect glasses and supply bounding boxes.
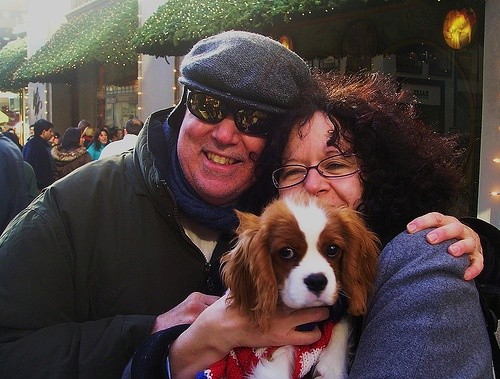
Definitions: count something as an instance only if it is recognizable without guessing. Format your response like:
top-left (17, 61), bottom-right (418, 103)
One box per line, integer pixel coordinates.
top-left (271, 154), bottom-right (362, 190)
top-left (186, 90), bottom-right (283, 136)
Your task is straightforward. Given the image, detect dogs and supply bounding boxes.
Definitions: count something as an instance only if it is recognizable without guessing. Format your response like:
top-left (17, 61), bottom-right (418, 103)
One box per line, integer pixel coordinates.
top-left (219, 190), bottom-right (383, 379)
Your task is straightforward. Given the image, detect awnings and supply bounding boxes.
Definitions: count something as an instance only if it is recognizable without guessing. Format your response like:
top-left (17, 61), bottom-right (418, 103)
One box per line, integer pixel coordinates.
top-left (132, 0), bottom-right (356, 57)
top-left (0, 37), bottom-right (28, 94)
top-left (13, 0), bottom-right (140, 84)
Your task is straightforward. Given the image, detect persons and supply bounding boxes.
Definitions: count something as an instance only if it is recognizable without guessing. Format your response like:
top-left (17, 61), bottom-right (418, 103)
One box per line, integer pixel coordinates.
top-left (121, 74), bottom-right (495, 379)
top-left (0, 30), bottom-right (500, 379)
top-left (0, 107), bottom-right (144, 236)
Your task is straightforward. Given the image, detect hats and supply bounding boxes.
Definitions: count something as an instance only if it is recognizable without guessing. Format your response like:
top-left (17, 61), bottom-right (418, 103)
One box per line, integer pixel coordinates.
top-left (179, 30), bottom-right (312, 117)
